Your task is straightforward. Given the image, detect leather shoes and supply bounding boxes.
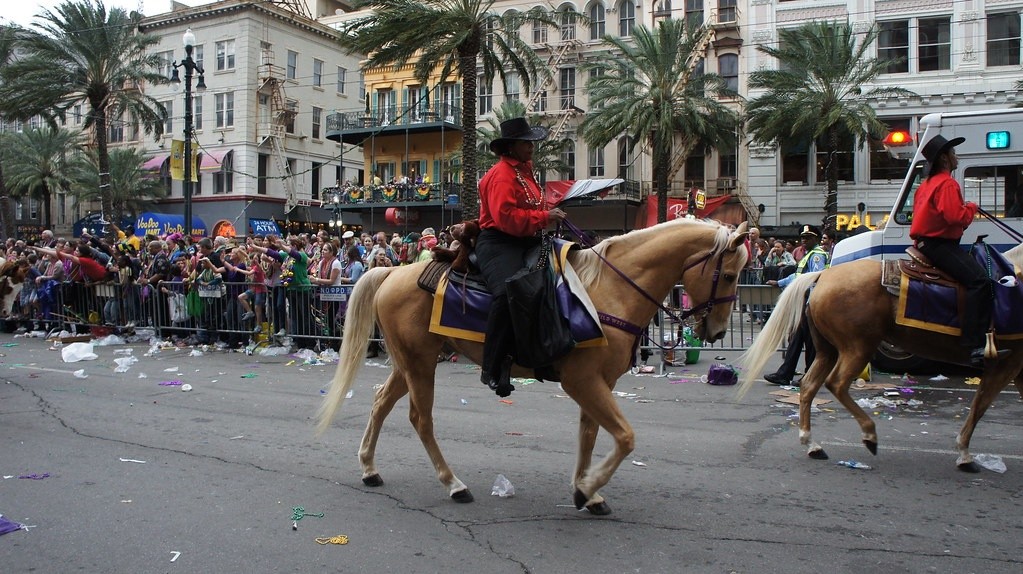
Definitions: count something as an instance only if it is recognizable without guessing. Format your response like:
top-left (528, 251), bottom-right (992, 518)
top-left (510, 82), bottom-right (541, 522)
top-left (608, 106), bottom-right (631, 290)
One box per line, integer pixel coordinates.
top-left (481, 371), bottom-right (515, 391)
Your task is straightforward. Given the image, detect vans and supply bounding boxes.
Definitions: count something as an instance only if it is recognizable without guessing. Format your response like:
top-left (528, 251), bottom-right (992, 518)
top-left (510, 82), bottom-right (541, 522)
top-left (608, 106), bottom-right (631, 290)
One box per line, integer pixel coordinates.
top-left (830, 109), bottom-right (1022, 373)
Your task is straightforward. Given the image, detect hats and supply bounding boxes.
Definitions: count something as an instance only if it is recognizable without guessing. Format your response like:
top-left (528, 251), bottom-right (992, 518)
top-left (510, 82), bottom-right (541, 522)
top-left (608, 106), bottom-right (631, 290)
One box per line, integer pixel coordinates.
top-left (341, 231), bottom-right (354, 238)
top-left (167, 233), bottom-right (183, 241)
top-left (921, 134), bottom-right (965, 176)
top-left (798, 224), bottom-right (822, 243)
top-left (490, 118), bottom-right (548, 154)
top-left (441, 225), bottom-right (450, 233)
top-left (123, 224), bottom-right (135, 234)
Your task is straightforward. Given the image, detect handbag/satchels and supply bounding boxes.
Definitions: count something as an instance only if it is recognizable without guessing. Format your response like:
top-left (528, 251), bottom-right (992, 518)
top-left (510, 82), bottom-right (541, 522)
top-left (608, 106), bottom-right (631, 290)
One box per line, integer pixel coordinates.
top-left (663, 332), bottom-right (687, 366)
top-left (167, 292), bottom-right (192, 323)
top-left (683, 328), bottom-right (701, 364)
top-left (187, 284), bottom-right (203, 316)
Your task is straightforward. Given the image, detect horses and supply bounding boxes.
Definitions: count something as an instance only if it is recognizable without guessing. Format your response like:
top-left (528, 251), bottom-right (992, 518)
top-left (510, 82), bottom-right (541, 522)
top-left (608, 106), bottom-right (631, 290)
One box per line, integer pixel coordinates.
top-left (312, 213), bottom-right (754, 515)
top-left (722, 241), bottom-right (1023, 473)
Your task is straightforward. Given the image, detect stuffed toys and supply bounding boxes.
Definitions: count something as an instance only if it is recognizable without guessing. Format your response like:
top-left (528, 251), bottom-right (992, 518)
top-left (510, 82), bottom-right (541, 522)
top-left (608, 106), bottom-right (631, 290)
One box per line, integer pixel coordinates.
top-left (429, 219), bottom-right (479, 271)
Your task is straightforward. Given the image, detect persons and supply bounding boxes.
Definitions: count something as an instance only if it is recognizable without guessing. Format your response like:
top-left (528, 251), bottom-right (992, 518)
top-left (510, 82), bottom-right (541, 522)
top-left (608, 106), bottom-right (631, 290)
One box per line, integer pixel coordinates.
top-left (0, 215), bottom-right (455, 359)
top-left (475, 117), bottom-right (567, 391)
top-left (909, 134), bottom-right (1013, 365)
top-left (740, 224), bottom-right (836, 385)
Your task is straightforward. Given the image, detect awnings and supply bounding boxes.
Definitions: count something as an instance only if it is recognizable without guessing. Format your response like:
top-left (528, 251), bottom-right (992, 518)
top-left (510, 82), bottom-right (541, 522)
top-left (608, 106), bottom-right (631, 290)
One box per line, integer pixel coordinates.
top-left (199, 149), bottom-right (233, 174)
top-left (136, 155), bottom-right (171, 178)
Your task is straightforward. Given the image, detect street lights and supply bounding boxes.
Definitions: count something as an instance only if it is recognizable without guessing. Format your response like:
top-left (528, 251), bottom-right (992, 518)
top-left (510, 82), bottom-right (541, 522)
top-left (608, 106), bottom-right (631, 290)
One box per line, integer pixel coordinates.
top-left (168, 28), bottom-right (210, 248)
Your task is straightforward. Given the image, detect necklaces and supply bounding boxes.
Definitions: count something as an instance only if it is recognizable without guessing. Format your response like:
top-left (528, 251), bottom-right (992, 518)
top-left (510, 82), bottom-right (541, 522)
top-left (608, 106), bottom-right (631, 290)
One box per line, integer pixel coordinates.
top-left (515, 167), bottom-right (546, 211)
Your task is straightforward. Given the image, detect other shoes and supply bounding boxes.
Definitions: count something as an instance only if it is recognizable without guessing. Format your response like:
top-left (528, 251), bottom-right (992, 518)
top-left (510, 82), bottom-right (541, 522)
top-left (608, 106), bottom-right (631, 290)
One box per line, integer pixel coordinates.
top-left (763, 373), bottom-right (789, 385)
top-left (278, 328), bottom-right (286, 335)
top-left (746, 318), bottom-right (757, 323)
top-left (242, 311), bottom-right (255, 320)
top-left (254, 324), bottom-right (262, 332)
top-left (7, 314), bottom-right (157, 337)
top-left (792, 380), bottom-right (800, 386)
top-left (648, 350), bottom-right (654, 355)
top-left (366, 352), bottom-right (378, 358)
top-left (971, 348), bottom-right (1012, 365)
